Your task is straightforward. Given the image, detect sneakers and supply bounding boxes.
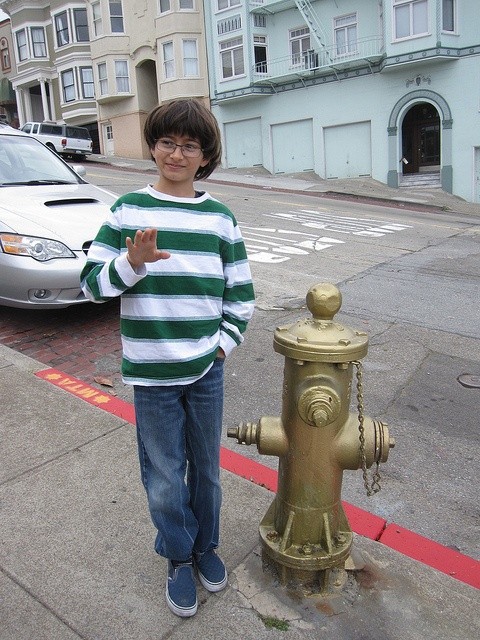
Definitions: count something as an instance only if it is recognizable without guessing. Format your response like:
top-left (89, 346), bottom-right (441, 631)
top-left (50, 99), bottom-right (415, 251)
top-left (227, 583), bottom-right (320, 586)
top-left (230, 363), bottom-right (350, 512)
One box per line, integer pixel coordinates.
top-left (165, 558), bottom-right (199, 618)
top-left (193, 548), bottom-right (228, 593)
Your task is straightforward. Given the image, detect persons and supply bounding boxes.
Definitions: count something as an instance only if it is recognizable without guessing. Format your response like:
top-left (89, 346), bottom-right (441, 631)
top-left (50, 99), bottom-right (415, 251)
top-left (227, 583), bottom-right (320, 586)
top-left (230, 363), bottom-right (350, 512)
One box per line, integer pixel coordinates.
top-left (79, 97), bottom-right (254, 619)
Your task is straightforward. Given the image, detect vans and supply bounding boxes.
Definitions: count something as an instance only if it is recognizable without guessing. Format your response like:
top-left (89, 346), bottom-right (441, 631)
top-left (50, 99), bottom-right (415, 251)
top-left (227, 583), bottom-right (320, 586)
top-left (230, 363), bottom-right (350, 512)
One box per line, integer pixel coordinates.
top-left (21, 121), bottom-right (93, 163)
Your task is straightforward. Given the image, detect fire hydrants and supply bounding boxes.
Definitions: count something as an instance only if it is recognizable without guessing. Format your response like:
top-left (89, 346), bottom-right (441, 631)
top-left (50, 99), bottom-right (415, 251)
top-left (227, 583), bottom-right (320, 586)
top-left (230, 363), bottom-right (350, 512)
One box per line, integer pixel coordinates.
top-left (229, 281), bottom-right (391, 585)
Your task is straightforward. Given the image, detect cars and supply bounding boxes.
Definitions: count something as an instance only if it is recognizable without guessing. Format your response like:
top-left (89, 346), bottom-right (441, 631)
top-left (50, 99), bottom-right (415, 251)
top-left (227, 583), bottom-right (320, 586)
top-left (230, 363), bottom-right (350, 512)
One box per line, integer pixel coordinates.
top-left (0, 120), bottom-right (134, 315)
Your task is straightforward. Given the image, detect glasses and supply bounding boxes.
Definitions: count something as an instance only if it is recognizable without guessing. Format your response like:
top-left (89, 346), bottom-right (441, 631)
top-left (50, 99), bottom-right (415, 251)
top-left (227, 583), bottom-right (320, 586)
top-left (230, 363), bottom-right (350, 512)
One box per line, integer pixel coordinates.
top-left (152, 138), bottom-right (204, 158)
